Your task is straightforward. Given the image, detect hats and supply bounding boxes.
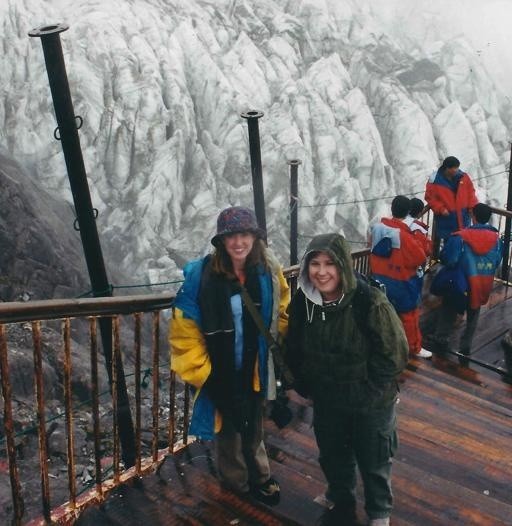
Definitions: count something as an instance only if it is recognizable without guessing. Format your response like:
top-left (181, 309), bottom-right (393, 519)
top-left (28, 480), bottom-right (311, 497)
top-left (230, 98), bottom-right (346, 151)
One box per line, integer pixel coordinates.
top-left (212, 207), bottom-right (266, 247)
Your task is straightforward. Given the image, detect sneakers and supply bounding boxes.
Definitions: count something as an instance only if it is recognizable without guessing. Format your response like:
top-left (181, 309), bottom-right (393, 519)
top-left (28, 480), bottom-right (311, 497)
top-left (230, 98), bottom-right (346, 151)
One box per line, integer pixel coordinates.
top-left (313, 493), bottom-right (335, 510)
top-left (416, 348), bottom-right (432, 359)
top-left (255, 478), bottom-right (279, 501)
top-left (368, 517), bottom-right (391, 526)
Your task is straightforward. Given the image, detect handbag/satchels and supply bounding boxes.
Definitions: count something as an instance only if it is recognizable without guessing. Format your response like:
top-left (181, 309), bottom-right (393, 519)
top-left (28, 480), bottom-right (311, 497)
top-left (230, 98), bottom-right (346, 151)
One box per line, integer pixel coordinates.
top-left (429, 265), bottom-right (467, 296)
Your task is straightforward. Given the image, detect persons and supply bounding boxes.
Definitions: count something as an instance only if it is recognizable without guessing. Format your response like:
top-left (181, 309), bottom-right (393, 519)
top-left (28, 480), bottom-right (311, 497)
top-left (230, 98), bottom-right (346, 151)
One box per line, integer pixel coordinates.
top-left (368, 195), bottom-right (433, 355)
top-left (284, 233), bottom-right (408, 525)
top-left (168, 206), bottom-right (292, 505)
top-left (442, 203), bottom-right (501, 365)
top-left (425, 156), bottom-right (481, 258)
top-left (403, 199), bottom-right (433, 291)
